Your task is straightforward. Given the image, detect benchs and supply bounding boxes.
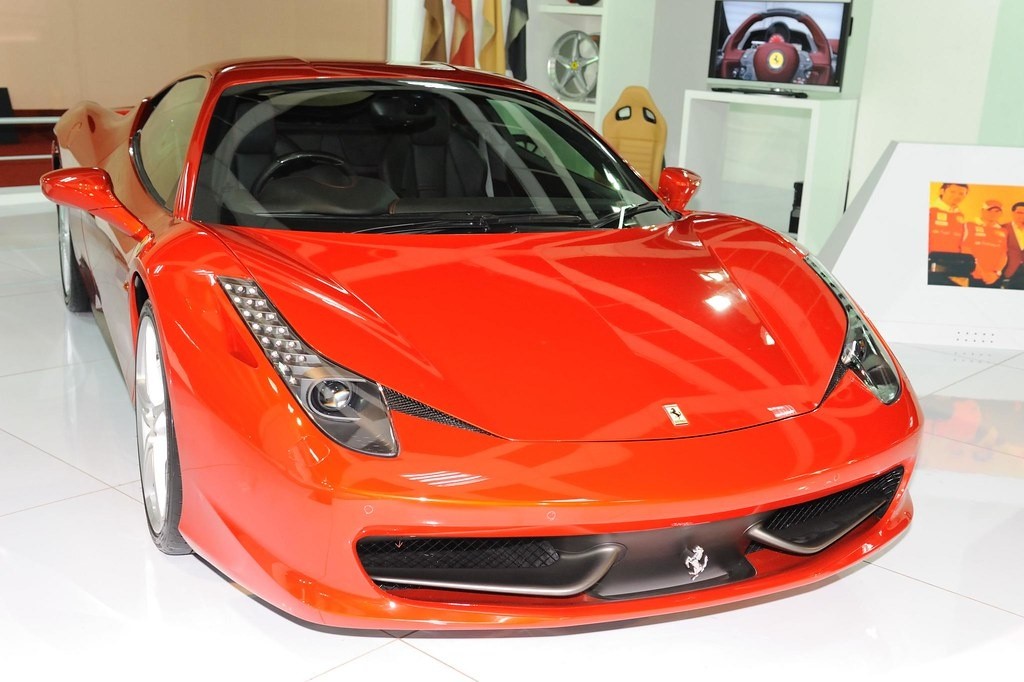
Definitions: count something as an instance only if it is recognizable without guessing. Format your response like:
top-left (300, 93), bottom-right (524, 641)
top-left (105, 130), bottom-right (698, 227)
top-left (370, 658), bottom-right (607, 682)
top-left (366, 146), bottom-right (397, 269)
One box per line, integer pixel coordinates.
top-left (276, 120), bottom-right (390, 177)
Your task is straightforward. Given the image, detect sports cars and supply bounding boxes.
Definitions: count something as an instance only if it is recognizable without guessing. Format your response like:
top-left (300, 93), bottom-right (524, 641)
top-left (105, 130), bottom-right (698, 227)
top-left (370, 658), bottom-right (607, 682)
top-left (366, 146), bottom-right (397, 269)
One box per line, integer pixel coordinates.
top-left (39, 56), bottom-right (926, 629)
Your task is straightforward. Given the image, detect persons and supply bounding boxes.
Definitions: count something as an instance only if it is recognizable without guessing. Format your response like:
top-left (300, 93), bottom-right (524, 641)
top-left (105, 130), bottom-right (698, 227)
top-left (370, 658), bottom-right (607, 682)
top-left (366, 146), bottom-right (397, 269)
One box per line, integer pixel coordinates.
top-left (928, 182), bottom-right (1024, 290)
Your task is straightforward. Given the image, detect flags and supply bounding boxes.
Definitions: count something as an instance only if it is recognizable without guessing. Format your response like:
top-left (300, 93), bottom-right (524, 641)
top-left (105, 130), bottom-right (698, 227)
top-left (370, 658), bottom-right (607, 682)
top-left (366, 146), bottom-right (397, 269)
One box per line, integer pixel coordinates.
top-left (479, 0), bottom-right (506, 76)
top-left (420, 0), bottom-right (446, 63)
top-left (449, 0), bottom-right (476, 67)
top-left (505, 0), bottom-right (529, 82)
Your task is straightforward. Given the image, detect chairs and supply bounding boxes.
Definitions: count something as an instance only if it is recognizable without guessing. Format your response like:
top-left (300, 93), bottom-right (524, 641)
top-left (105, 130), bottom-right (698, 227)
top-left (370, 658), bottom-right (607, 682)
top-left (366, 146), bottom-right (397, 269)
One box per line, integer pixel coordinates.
top-left (379, 115), bottom-right (489, 199)
top-left (215, 102), bottom-right (304, 224)
